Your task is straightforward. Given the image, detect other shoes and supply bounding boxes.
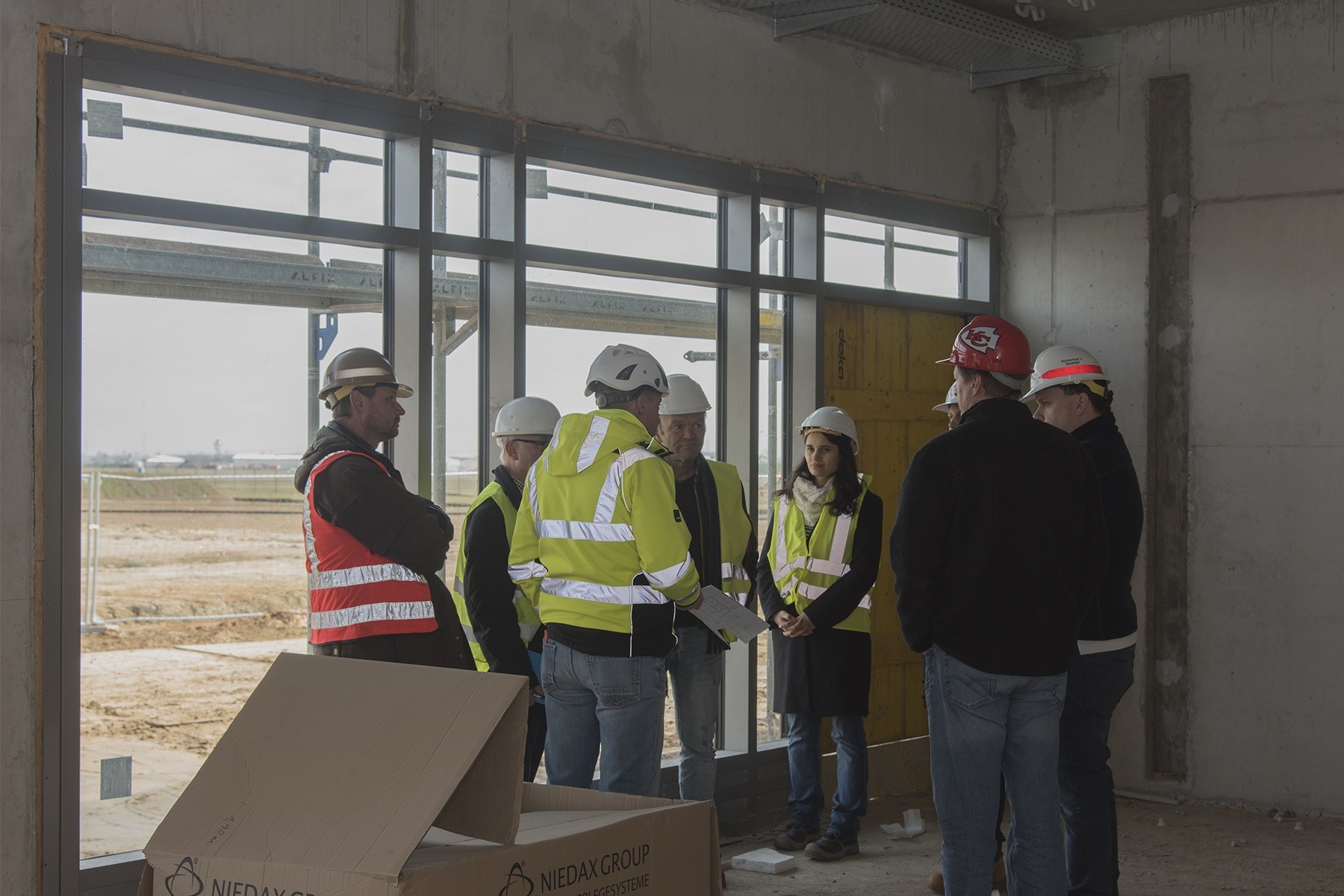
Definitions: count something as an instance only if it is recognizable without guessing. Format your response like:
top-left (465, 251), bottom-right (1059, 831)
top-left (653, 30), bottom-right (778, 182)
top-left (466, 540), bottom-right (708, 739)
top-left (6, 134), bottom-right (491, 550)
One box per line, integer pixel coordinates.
top-left (928, 851), bottom-right (1005, 895)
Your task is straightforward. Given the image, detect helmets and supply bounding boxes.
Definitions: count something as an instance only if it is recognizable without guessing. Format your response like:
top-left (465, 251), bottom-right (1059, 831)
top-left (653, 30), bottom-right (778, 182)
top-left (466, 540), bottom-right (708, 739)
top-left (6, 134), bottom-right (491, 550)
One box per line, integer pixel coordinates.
top-left (1019, 346), bottom-right (1111, 403)
top-left (935, 316), bottom-right (1035, 374)
top-left (658, 374), bottom-right (712, 414)
top-left (491, 396), bottom-right (560, 437)
top-left (584, 344), bottom-right (670, 397)
top-left (795, 406), bottom-right (859, 455)
top-left (318, 347), bottom-right (414, 397)
top-left (932, 381), bottom-right (959, 412)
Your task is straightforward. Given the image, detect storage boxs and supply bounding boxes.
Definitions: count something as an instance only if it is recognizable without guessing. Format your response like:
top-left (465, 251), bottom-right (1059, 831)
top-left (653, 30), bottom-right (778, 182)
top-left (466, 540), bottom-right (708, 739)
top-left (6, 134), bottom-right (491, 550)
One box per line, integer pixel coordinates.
top-left (138, 651), bottom-right (722, 896)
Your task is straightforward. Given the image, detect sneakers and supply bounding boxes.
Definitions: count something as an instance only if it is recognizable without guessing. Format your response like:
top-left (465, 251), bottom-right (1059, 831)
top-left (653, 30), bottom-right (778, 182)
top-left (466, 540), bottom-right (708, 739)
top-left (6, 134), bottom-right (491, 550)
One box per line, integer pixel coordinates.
top-left (805, 832), bottom-right (859, 862)
top-left (774, 822), bottom-right (820, 850)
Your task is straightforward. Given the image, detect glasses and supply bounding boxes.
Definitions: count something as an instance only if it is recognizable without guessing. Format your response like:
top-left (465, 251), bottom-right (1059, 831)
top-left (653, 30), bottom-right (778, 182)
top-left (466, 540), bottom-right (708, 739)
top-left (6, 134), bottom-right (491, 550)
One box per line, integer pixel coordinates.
top-left (511, 438), bottom-right (550, 453)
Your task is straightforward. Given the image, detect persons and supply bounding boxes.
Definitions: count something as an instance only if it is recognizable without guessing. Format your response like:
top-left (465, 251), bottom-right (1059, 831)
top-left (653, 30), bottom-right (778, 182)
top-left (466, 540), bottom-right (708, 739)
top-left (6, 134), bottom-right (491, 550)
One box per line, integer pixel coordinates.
top-left (294, 346), bottom-right (454, 667)
top-left (757, 405), bottom-right (882, 860)
top-left (508, 343), bottom-right (704, 800)
top-left (649, 375), bottom-right (759, 893)
top-left (453, 396), bottom-right (562, 784)
top-left (1017, 345), bottom-right (1146, 896)
top-left (927, 373), bottom-right (1009, 896)
top-left (889, 315), bottom-right (1110, 895)
